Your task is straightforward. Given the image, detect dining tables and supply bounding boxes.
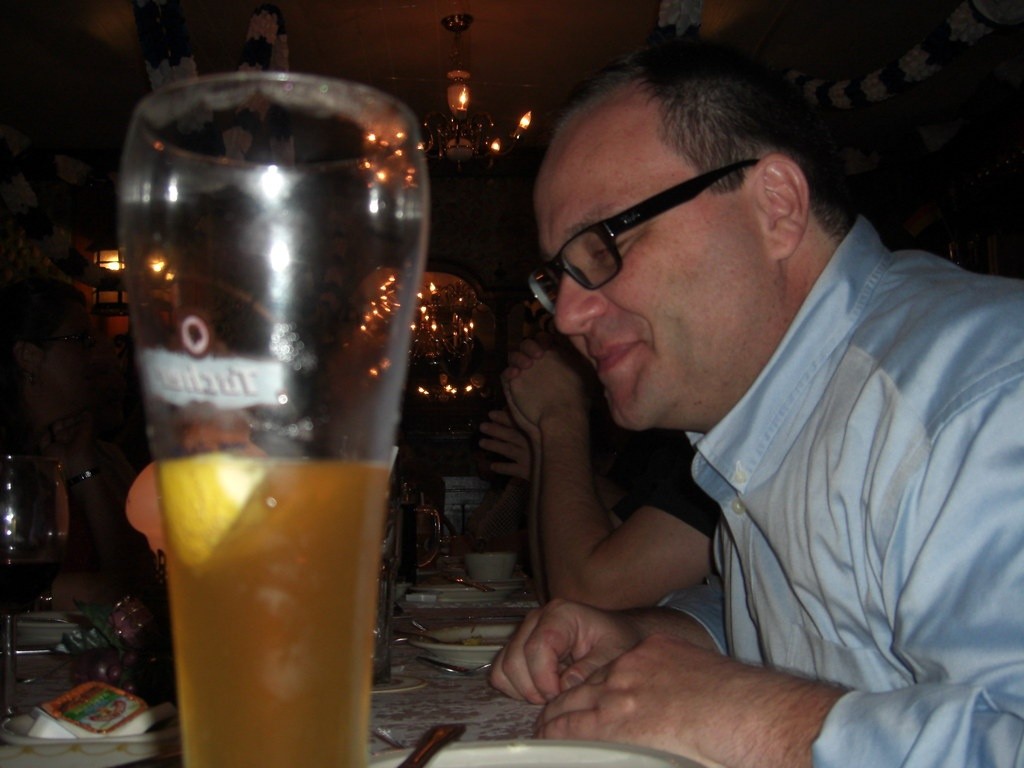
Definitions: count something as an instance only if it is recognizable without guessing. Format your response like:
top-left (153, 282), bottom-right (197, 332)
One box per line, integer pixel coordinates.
top-left (0, 552), bottom-right (725, 768)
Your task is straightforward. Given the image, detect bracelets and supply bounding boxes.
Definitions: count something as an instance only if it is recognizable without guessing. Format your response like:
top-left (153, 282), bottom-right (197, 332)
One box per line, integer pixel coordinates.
top-left (65, 466), bottom-right (101, 492)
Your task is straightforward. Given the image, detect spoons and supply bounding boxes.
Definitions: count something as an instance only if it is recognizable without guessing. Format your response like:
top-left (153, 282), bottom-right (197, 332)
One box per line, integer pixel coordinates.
top-left (417, 655), bottom-right (493, 676)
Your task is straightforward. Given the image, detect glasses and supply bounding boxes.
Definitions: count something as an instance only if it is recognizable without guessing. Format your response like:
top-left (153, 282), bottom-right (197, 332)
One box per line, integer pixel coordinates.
top-left (528, 157), bottom-right (762, 316)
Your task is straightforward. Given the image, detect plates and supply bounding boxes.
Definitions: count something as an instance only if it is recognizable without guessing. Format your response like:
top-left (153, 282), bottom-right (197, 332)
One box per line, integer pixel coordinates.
top-left (367, 739), bottom-right (707, 768)
top-left (406, 621), bottom-right (516, 661)
top-left (404, 587), bottom-right (515, 606)
top-left (462, 577), bottom-right (524, 590)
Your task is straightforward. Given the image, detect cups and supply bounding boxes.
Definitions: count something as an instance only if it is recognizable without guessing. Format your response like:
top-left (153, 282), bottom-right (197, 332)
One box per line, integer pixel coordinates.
top-left (369, 508), bottom-right (401, 686)
top-left (121, 69), bottom-right (428, 766)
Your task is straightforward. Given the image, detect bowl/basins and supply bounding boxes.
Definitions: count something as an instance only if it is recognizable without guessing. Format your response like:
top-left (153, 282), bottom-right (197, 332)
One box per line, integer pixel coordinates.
top-left (465, 552), bottom-right (516, 580)
top-left (15, 611), bottom-right (85, 639)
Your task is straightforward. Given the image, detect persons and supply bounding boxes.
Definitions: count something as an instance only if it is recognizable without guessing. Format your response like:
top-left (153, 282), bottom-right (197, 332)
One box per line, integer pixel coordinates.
top-left (0, 275), bottom-right (148, 617)
top-left (479, 338), bottom-right (720, 610)
top-left (487, 42), bottom-right (1023, 767)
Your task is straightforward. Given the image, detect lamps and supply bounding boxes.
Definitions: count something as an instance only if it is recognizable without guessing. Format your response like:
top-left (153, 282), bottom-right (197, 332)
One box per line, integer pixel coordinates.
top-left (415, 15), bottom-right (532, 165)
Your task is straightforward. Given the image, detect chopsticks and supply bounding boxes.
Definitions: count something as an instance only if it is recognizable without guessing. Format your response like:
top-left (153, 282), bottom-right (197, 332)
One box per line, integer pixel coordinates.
top-left (0, 715), bottom-right (178, 745)
